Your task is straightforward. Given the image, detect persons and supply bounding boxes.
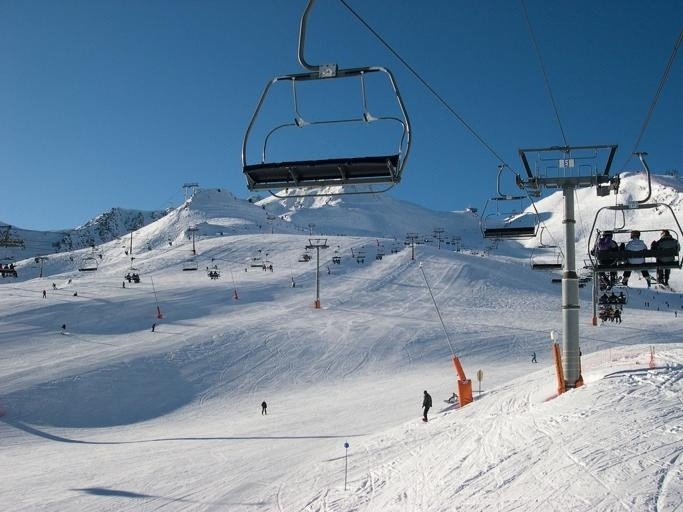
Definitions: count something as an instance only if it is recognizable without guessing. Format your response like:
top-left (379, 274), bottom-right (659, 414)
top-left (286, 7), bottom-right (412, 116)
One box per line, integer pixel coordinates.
top-left (599, 276), bottom-right (626, 324)
top-left (61, 324), bottom-right (65, 335)
top-left (591, 231), bottom-right (619, 285)
top-left (152, 321), bottom-right (157, 332)
top-left (262, 401), bottom-right (267, 415)
top-left (126, 272), bottom-right (140, 284)
top-left (532, 352), bottom-right (537, 363)
top-left (123, 281), bottom-right (125, 287)
top-left (52, 282), bottom-right (57, 290)
top-left (622, 231), bottom-right (652, 286)
top-left (651, 230), bottom-right (680, 284)
top-left (422, 391), bottom-right (432, 422)
top-left (42, 290), bottom-right (47, 299)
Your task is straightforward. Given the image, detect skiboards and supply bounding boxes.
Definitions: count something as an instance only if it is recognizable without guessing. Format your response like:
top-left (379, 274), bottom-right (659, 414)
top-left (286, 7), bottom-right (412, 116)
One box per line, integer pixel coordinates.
top-left (621, 278), bottom-right (651, 287)
top-left (649, 275), bottom-right (675, 292)
top-left (599, 277), bottom-right (622, 291)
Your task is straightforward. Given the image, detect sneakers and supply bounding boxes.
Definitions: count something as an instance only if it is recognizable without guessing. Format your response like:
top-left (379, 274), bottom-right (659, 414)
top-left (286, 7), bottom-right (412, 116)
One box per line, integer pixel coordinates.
top-left (646, 276), bottom-right (668, 288)
top-left (603, 276), bottom-right (628, 289)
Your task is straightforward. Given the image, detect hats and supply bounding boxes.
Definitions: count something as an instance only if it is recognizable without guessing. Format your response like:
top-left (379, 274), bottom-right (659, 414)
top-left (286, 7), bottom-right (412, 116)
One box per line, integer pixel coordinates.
top-left (603, 231), bottom-right (612, 238)
top-left (631, 232), bottom-right (640, 237)
